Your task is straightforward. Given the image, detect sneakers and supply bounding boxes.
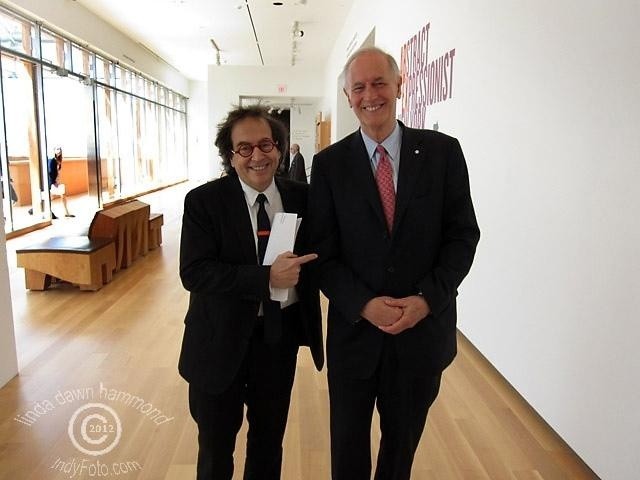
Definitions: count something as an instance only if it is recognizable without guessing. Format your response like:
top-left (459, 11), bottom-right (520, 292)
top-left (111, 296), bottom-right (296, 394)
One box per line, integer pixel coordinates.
top-left (64, 212), bottom-right (75, 217)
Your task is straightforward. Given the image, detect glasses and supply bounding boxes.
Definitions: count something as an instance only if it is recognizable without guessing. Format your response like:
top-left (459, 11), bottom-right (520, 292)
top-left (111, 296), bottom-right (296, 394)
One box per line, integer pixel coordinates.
top-left (231, 140), bottom-right (277, 157)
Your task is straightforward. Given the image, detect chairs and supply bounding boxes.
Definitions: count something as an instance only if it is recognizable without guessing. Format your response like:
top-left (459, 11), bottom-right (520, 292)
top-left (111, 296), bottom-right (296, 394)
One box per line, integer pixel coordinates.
top-left (78, 197), bottom-right (150, 272)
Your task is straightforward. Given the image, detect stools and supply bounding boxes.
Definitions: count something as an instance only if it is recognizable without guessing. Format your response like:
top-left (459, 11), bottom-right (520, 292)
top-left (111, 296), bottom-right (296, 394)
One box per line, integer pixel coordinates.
top-left (148, 213), bottom-right (163, 251)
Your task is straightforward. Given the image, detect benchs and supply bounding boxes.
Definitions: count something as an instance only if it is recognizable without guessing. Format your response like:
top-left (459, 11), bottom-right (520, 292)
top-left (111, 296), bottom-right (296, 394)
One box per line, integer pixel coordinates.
top-left (16, 236), bottom-right (117, 292)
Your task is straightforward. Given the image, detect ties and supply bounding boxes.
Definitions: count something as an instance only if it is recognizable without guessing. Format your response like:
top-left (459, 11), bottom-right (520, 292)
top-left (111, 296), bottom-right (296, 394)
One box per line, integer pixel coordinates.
top-left (376, 143), bottom-right (396, 234)
top-left (256, 193), bottom-right (271, 270)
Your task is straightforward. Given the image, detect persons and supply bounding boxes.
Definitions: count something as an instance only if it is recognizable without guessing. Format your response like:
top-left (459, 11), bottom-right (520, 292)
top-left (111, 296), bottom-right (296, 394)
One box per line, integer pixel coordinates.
top-left (287, 144), bottom-right (307, 184)
top-left (178, 104), bottom-right (324, 480)
top-left (47, 148), bottom-right (75, 219)
top-left (312, 47), bottom-right (480, 480)
